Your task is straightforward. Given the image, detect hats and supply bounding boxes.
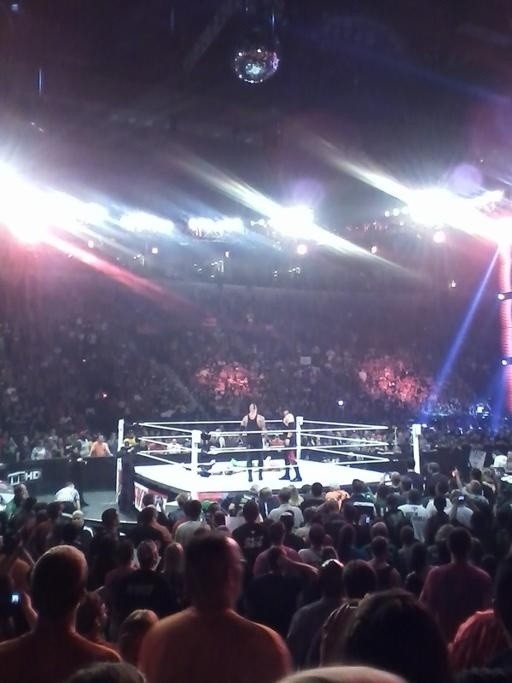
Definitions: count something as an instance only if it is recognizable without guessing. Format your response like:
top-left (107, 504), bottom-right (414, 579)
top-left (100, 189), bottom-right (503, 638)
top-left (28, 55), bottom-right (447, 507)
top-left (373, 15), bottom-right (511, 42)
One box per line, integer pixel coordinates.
top-left (278, 509), bottom-right (294, 528)
top-left (492, 455), bottom-right (508, 470)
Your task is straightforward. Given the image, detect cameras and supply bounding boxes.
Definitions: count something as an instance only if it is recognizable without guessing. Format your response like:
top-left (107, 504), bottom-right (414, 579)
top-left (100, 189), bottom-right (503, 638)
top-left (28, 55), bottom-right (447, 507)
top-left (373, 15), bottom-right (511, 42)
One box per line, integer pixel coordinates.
top-left (11, 593), bottom-right (21, 606)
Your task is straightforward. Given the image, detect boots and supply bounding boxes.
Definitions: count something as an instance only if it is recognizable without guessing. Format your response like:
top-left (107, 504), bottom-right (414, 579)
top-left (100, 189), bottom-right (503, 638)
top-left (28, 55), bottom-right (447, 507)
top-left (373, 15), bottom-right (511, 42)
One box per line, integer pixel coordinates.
top-left (258, 468), bottom-right (262, 480)
top-left (278, 466), bottom-right (291, 481)
top-left (248, 469), bottom-right (252, 482)
top-left (290, 466), bottom-right (302, 481)
